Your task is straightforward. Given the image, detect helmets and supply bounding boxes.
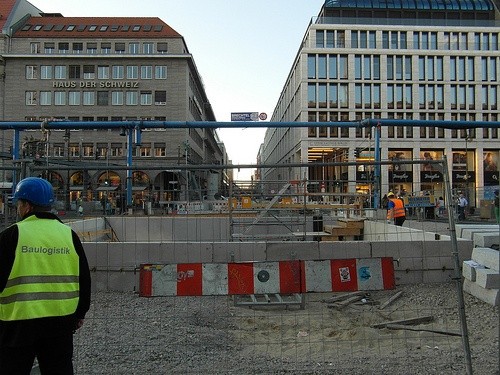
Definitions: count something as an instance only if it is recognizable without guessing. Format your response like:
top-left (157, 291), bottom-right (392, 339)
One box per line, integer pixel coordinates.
top-left (387, 192), bottom-right (394, 198)
top-left (13, 177), bottom-right (55, 207)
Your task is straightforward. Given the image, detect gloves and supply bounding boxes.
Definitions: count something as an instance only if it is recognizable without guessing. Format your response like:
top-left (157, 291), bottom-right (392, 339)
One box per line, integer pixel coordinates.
top-left (387, 220), bottom-right (390, 224)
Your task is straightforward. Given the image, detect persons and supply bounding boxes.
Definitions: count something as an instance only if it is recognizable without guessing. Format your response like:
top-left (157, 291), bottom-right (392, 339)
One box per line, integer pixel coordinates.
top-left (100, 192), bottom-right (120, 215)
top-left (356, 187), bottom-right (437, 222)
top-left (78, 204), bottom-right (83, 215)
top-left (0, 178), bottom-right (92, 372)
top-left (437, 196), bottom-right (445, 216)
top-left (491, 191), bottom-right (500, 225)
top-left (386, 192), bottom-right (406, 226)
top-left (457, 193), bottom-right (466, 221)
